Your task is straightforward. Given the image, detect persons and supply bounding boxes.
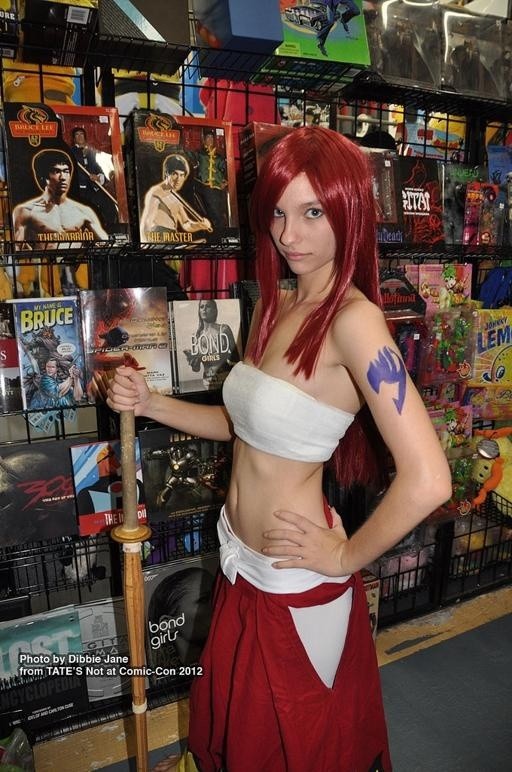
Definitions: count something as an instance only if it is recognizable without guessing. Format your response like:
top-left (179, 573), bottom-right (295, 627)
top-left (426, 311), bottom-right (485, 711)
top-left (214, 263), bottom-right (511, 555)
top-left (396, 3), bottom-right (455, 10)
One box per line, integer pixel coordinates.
top-left (20, 323), bottom-right (65, 363)
top-left (140, 153), bottom-right (214, 246)
top-left (146, 566), bottom-right (216, 671)
top-left (30, 356), bottom-right (79, 416)
top-left (8, 150), bottom-right (106, 248)
top-left (187, 131), bottom-right (226, 187)
top-left (108, 127), bottom-right (452, 772)
top-left (72, 127), bottom-right (107, 207)
top-left (184, 300), bottom-right (240, 388)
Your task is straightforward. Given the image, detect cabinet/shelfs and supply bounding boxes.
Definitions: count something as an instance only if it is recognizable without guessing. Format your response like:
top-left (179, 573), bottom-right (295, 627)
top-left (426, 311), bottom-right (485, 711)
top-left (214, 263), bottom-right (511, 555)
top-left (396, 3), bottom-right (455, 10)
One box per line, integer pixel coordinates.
top-left (0, 0), bottom-right (512, 772)
